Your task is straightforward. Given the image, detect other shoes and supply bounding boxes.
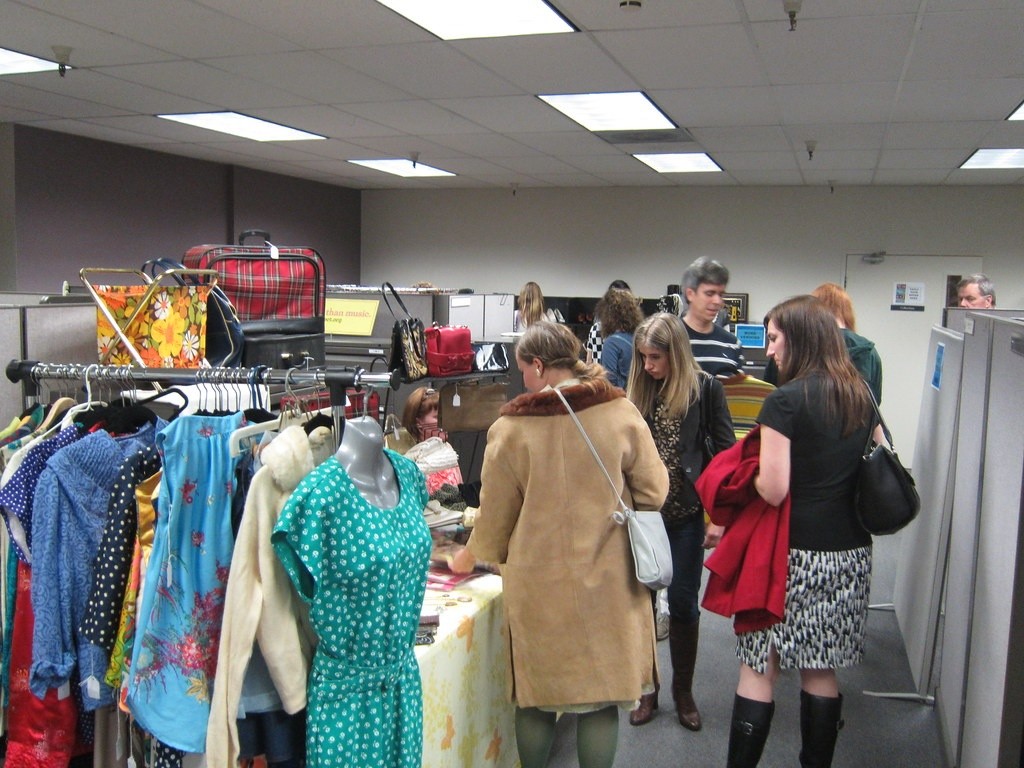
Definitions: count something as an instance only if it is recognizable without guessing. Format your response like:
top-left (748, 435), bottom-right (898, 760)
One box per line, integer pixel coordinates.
top-left (657, 611), bottom-right (670, 639)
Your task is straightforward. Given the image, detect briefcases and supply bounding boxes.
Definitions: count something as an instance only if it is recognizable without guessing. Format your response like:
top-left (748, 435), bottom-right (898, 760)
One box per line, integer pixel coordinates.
top-left (242, 333), bottom-right (327, 371)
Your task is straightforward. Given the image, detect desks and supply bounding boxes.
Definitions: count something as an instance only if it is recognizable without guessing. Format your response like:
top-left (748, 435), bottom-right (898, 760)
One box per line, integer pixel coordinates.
top-left (409, 561), bottom-right (510, 768)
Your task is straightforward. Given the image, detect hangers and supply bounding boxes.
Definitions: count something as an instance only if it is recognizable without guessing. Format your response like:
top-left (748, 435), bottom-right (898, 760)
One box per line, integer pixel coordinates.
top-left (0, 362), bottom-right (383, 478)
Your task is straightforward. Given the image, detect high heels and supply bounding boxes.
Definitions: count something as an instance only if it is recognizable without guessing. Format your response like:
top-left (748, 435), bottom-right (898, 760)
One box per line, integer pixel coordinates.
top-left (671, 682), bottom-right (701, 732)
top-left (629, 680), bottom-right (660, 725)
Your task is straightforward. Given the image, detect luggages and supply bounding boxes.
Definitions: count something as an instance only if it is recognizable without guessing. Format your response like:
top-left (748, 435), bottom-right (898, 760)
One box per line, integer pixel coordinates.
top-left (281, 389), bottom-right (380, 429)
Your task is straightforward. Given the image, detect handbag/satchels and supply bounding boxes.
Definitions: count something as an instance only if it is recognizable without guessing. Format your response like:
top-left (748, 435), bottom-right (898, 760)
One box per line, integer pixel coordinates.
top-left (471, 341), bottom-right (510, 373)
top-left (611, 506), bottom-right (673, 591)
top-left (383, 413), bottom-right (417, 455)
top-left (422, 320), bottom-right (476, 377)
top-left (181, 229), bottom-right (327, 335)
top-left (854, 376), bottom-right (921, 536)
top-left (438, 379), bottom-right (510, 432)
top-left (141, 257), bottom-right (244, 368)
top-left (381, 282), bottom-right (428, 383)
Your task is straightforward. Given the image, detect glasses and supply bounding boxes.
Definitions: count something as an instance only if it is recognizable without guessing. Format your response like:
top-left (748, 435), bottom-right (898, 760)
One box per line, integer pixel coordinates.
top-left (421, 388), bottom-right (437, 401)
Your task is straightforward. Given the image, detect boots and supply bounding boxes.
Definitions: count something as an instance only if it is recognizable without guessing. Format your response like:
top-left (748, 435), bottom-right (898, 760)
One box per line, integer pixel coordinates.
top-left (727, 690), bottom-right (775, 768)
top-left (799, 688), bottom-right (845, 768)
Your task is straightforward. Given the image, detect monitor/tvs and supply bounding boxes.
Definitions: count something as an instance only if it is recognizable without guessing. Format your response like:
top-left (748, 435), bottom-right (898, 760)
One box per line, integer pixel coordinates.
top-left (735, 324), bottom-right (765, 349)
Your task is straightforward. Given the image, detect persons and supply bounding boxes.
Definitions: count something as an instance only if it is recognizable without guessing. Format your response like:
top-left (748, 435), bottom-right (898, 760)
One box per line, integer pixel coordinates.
top-left (763, 283), bottom-right (883, 408)
top-left (586, 255), bottom-right (745, 731)
top-left (450, 320), bottom-right (669, 768)
top-left (402, 387), bottom-right (448, 446)
top-left (513, 282), bottom-right (549, 333)
top-left (291, 415), bottom-right (432, 768)
top-left (954, 272), bottom-right (996, 310)
top-left (694, 295), bottom-right (883, 768)
